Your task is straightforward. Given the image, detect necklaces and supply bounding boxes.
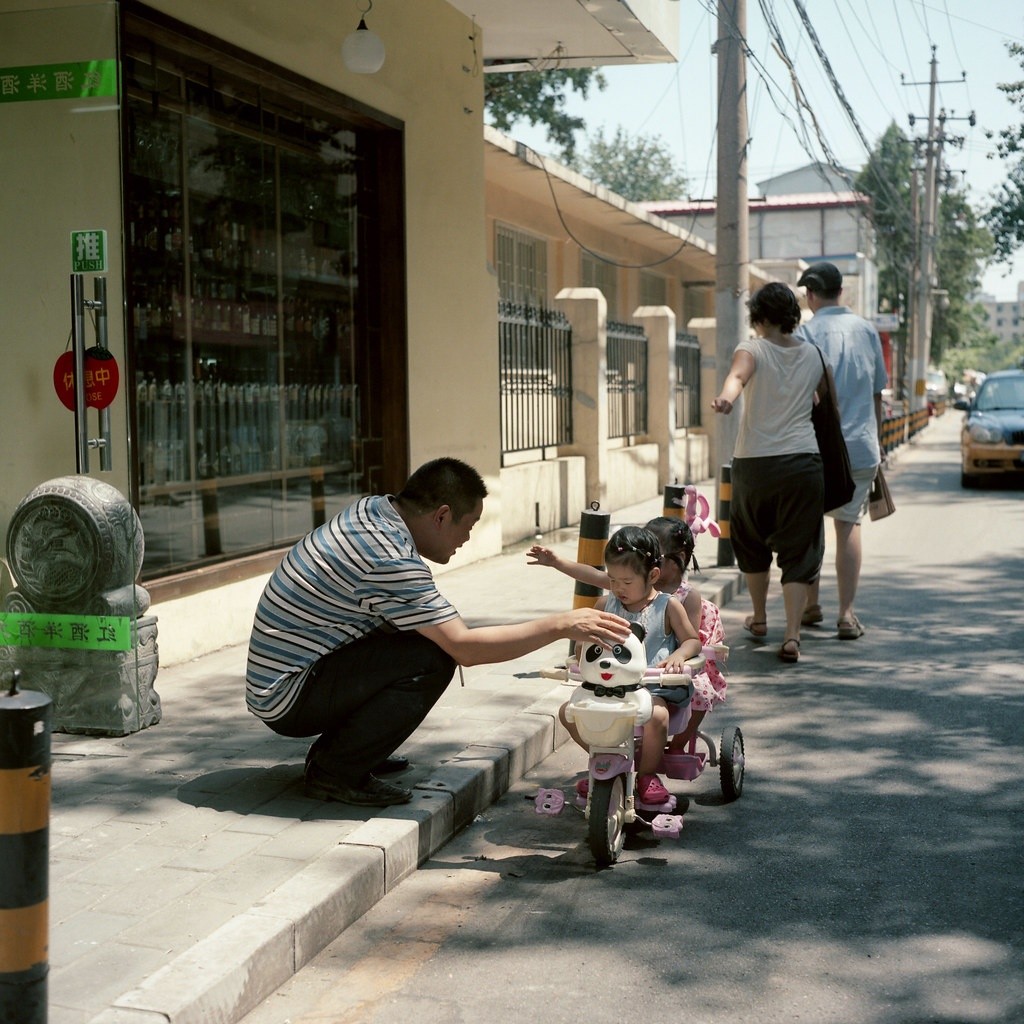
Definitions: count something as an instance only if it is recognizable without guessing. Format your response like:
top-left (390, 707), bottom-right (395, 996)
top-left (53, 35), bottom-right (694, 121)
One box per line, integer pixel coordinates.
top-left (621, 590), bottom-right (659, 613)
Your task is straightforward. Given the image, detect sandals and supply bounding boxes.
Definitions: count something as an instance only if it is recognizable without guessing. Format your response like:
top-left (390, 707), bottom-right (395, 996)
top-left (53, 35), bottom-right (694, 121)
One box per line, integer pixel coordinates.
top-left (836, 614), bottom-right (865, 638)
top-left (801, 604), bottom-right (824, 624)
top-left (743, 616), bottom-right (767, 636)
top-left (781, 639), bottom-right (801, 660)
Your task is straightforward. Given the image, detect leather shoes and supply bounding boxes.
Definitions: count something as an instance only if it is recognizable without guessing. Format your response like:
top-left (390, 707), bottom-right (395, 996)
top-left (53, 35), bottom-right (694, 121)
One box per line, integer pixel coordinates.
top-left (304, 769), bottom-right (414, 805)
top-left (375, 754), bottom-right (410, 770)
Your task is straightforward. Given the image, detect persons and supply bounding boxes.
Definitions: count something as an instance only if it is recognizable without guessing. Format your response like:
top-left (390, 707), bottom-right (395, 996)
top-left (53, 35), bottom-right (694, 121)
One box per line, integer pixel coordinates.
top-left (524, 517), bottom-right (728, 777)
top-left (709, 282), bottom-right (837, 662)
top-left (244, 458), bottom-right (633, 806)
top-left (796, 262), bottom-right (887, 640)
top-left (558, 526), bottom-right (703, 805)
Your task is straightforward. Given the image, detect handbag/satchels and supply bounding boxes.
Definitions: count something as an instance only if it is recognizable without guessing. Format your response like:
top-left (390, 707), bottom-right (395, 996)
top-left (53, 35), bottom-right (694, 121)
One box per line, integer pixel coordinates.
top-left (811, 344), bottom-right (856, 513)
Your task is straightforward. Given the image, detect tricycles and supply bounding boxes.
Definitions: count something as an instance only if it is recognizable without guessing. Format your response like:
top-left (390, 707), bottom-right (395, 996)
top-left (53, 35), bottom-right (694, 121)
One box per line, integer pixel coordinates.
top-left (511, 479), bottom-right (747, 866)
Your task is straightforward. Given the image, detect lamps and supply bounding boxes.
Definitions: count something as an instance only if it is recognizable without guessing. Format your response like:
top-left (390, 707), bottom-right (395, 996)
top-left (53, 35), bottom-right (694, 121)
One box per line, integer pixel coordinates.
top-left (339, 0), bottom-right (385, 73)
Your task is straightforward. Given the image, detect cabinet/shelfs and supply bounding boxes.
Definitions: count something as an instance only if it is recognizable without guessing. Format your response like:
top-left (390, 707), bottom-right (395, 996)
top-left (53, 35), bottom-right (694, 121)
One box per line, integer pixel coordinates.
top-left (123, 99), bottom-right (362, 374)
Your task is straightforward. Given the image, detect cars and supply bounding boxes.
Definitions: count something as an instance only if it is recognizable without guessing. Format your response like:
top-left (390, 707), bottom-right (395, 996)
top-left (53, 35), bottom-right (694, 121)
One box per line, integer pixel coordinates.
top-left (926, 368), bottom-right (951, 417)
top-left (951, 367), bottom-right (1024, 491)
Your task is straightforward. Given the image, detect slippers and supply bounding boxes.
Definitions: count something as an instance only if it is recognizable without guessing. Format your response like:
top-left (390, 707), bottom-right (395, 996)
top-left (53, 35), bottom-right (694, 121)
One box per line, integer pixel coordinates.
top-left (637, 774), bottom-right (670, 804)
top-left (577, 778), bottom-right (589, 798)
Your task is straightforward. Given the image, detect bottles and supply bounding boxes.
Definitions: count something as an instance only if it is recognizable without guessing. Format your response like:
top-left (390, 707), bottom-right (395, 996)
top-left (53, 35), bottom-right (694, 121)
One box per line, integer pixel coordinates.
top-left (123, 200), bottom-right (351, 338)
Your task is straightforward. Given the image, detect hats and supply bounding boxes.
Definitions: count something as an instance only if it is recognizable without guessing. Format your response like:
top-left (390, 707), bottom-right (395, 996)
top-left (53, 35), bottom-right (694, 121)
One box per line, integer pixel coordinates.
top-left (797, 262), bottom-right (842, 290)
